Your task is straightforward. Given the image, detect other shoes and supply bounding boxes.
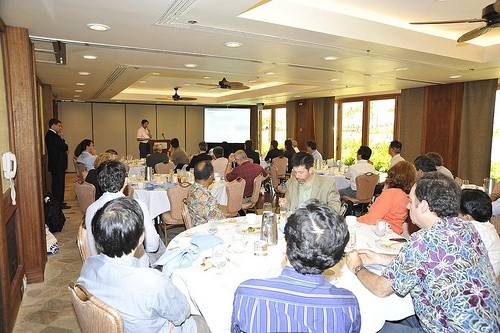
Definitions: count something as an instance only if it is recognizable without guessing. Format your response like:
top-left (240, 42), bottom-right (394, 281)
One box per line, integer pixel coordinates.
top-left (340, 204), bottom-right (348, 216)
top-left (60, 203), bottom-right (71, 209)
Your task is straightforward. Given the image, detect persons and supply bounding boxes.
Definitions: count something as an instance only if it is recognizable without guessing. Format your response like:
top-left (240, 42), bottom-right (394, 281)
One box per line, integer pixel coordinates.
top-left (45, 119), bottom-right (72, 209)
top-left (76, 119), bottom-right (500, 333)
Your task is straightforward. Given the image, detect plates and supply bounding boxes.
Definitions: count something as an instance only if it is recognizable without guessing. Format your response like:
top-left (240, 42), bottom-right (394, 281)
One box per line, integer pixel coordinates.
top-left (366, 240), bottom-right (403, 255)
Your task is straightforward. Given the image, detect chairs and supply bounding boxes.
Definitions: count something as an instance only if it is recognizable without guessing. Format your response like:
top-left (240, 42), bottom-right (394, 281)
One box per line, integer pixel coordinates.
top-left (67, 154), bottom-right (379, 333)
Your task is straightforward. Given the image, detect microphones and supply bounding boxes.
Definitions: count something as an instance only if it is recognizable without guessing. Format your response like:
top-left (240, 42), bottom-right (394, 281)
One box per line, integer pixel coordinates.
top-left (162, 133), bottom-right (165, 140)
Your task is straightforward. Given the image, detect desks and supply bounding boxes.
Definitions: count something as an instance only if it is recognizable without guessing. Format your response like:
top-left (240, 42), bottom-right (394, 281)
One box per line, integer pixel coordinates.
top-left (151, 213), bottom-right (415, 333)
top-left (129, 173), bottom-right (227, 253)
top-left (319, 166), bottom-right (388, 190)
top-left (120, 159), bottom-right (147, 175)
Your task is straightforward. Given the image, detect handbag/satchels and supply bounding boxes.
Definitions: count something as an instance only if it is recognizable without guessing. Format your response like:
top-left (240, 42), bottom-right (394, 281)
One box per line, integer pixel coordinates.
top-left (43, 192), bottom-right (67, 233)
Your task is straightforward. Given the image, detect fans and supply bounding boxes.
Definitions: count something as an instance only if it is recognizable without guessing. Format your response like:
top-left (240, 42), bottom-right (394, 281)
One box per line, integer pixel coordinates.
top-left (195, 77), bottom-right (250, 90)
top-left (155, 88), bottom-right (197, 102)
top-left (408, 0), bottom-right (500, 43)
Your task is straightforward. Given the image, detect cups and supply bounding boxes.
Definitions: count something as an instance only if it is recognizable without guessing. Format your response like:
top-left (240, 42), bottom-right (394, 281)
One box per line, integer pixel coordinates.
top-left (313, 158), bottom-right (349, 175)
top-left (345, 215), bottom-right (358, 248)
top-left (462, 178), bottom-right (494, 197)
top-left (254, 200), bottom-right (289, 257)
top-left (132, 166), bottom-right (194, 183)
top-left (375, 219), bottom-right (391, 236)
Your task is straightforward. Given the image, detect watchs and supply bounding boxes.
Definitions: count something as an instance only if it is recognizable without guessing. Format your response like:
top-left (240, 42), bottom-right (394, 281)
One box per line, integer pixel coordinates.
top-left (353, 266), bottom-right (365, 275)
